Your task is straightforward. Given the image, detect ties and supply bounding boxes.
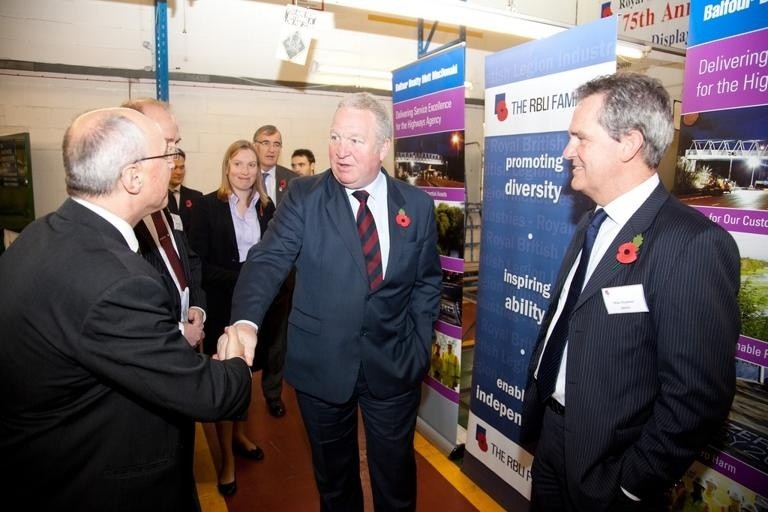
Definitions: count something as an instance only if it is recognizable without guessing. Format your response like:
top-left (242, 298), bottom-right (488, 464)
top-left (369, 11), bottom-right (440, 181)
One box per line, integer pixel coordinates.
top-left (152, 211), bottom-right (188, 291)
top-left (535, 209), bottom-right (609, 403)
top-left (353, 188), bottom-right (382, 293)
top-left (261, 172), bottom-right (269, 195)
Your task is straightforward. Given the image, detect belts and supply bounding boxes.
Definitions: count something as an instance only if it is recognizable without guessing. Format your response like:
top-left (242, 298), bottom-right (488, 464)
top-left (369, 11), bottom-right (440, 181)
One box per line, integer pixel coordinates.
top-left (546, 397), bottom-right (565, 418)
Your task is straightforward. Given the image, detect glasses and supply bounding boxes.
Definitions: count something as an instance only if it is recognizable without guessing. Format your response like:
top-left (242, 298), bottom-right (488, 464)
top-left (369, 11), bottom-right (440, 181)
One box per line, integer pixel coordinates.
top-left (255, 139), bottom-right (282, 149)
top-left (133, 146), bottom-right (179, 165)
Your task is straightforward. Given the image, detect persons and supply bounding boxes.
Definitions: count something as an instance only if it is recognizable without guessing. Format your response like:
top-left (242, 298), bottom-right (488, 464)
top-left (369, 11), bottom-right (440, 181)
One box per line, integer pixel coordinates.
top-left (216, 90), bottom-right (444, 512)
top-left (0, 106), bottom-right (254, 512)
top-left (290, 148), bottom-right (316, 178)
top-left (249, 124), bottom-right (303, 418)
top-left (187, 139), bottom-right (275, 499)
top-left (519, 71), bottom-right (741, 511)
top-left (165, 146), bottom-right (204, 219)
top-left (430, 338), bottom-right (460, 391)
top-left (120, 97), bottom-right (203, 512)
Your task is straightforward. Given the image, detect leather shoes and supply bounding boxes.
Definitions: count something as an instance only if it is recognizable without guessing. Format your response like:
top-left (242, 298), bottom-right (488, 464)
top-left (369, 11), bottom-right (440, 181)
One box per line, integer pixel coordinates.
top-left (233, 439), bottom-right (264, 460)
top-left (266, 398), bottom-right (285, 417)
top-left (219, 473), bottom-right (237, 496)
